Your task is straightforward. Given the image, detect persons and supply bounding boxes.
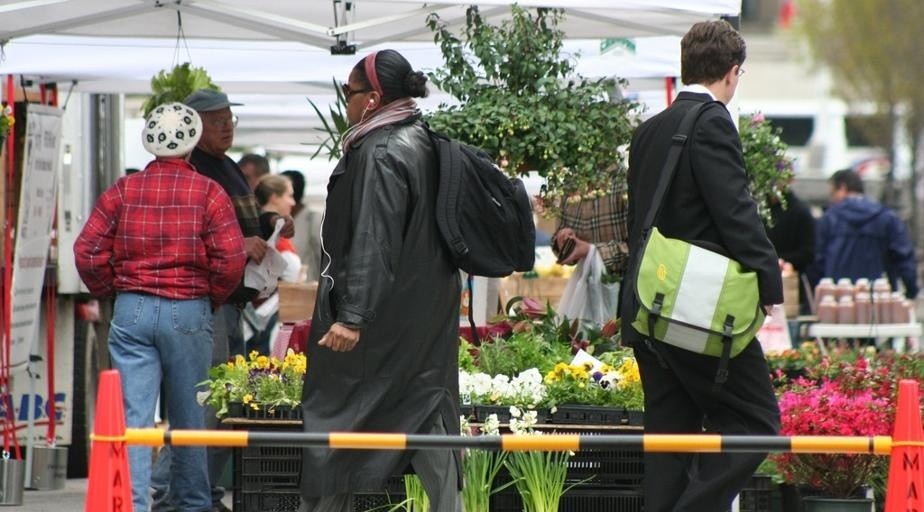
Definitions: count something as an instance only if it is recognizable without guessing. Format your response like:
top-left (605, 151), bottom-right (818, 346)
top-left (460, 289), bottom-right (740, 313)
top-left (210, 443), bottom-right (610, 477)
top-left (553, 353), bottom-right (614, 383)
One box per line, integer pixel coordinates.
top-left (748, 143), bottom-right (918, 347)
top-left (294, 46), bottom-right (468, 510)
top-left (151, 91), bottom-right (322, 512)
top-left (614, 12), bottom-right (787, 512)
top-left (72, 99), bottom-right (250, 512)
top-left (549, 166), bottom-right (627, 320)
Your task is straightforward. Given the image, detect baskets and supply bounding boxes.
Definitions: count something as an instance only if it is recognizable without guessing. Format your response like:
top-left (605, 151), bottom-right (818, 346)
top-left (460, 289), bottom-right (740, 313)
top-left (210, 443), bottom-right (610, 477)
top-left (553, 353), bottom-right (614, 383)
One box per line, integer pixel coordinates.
top-left (225, 405), bottom-right (415, 509)
top-left (225, 403), bottom-right (786, 509)
top-left (739, 476), bottom-right (784, 509)
top-left (460, 405), bottom-right (646, 510)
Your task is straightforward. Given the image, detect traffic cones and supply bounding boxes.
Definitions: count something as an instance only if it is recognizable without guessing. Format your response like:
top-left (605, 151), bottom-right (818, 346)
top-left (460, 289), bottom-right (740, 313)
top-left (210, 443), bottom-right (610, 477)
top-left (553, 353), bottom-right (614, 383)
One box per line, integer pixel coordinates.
top-left (883, 378), bottom-right (923, 512)
top-left (84, 369), bottom-right (131, 511)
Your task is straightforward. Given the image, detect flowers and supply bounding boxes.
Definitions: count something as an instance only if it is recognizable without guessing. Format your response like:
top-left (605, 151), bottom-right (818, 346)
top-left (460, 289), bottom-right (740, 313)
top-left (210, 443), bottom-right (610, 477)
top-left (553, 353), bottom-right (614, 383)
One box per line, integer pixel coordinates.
top-left (191, 321), bottom-right (921, 497)
top-left (736, 112), bottom-right (799, 232)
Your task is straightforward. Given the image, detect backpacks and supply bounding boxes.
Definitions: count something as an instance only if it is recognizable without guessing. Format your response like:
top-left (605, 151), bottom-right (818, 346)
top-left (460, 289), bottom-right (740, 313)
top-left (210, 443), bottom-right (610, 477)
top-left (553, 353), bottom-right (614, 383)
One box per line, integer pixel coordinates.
top-left (414, 123), bottom-right (535, 280)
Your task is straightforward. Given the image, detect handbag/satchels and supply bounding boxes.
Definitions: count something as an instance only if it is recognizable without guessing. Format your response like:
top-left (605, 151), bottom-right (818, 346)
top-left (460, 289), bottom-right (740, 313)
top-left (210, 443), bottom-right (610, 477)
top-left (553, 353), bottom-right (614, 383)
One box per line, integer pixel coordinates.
top-left (627, 224), bottom-right (766, 361)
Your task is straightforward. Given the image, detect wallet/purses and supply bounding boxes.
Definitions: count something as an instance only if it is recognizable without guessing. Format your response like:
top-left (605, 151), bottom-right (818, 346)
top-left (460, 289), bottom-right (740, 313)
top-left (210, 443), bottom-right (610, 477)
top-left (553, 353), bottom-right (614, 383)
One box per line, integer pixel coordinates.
top-left (557, 238), bottom-right (576, 261)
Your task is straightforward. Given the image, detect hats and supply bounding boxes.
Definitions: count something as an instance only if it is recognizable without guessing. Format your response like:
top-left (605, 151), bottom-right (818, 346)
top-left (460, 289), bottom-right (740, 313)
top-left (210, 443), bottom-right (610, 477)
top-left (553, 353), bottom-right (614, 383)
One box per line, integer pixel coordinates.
top-left (140, 101), bottom-right (204, 158)
top-left (182, 88), bottom-right (245, 113)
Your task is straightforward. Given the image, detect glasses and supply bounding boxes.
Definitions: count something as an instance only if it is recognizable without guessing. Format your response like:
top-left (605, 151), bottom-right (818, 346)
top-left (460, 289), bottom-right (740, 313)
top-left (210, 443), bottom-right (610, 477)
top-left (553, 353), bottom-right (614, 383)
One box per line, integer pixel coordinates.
top-left (737, 67), bottom-right (744, 77)
top-left (199, 114), bottom-right (239, 130)
top-left (341, 82), bottom-right (372, 102)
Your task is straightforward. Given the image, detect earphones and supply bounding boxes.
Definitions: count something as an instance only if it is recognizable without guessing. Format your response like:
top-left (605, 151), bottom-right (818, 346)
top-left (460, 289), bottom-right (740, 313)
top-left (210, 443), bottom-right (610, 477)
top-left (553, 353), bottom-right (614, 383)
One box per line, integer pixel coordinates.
top-left (369, 98), bottom-right (374, 104)
